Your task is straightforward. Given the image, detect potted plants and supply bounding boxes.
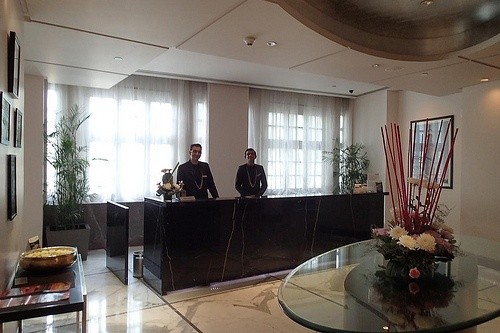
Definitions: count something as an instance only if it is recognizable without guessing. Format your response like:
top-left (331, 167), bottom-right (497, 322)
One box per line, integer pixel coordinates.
top-left (43, 104), bottom-right (109, 259)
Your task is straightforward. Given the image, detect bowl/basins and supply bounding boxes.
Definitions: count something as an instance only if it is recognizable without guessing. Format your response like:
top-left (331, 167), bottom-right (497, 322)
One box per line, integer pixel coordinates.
top-left (19, 245), bottom-right (78, 271)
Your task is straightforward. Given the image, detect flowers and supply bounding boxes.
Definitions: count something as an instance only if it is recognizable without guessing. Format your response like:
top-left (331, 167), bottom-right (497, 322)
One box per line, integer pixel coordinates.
top-left (370, 177), bottom-right (461, 278)
top-left (373, 265), bottom-right (464, 333)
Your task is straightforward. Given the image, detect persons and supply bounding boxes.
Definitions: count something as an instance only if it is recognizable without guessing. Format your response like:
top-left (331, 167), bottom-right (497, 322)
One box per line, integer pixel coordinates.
top-left (175, 142), bottom-right (221, 199)
top-left (233, 147), bottom-right (269, 199)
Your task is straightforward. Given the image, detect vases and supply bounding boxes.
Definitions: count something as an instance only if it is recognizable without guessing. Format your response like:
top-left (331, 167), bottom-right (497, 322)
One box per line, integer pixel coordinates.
top-left (385, 260), bottom-right (430, 279)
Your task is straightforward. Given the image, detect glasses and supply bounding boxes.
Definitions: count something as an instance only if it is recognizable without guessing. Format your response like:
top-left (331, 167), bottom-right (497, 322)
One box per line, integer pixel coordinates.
top-left (190, 150), bottom-right (202, 154)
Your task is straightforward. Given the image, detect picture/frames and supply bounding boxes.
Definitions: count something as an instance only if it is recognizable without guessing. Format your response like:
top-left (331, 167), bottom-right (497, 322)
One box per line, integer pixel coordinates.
top-left (410, 115), bottom-right (454, 189)
top-left (10, 154), bottom-right (17, 220)
top-left (8, 31), bottom-right (21, 99)
top-left (0, 91), bottom-right (11, 145)
top-left (14, 108), bottom-right (23, 148)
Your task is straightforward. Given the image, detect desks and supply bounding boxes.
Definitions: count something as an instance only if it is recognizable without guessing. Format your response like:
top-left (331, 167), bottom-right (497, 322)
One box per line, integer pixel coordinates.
top-left (278, 233), bottom-right (500, 333)
top-left (0, 247), bottom-right (87, 333)
